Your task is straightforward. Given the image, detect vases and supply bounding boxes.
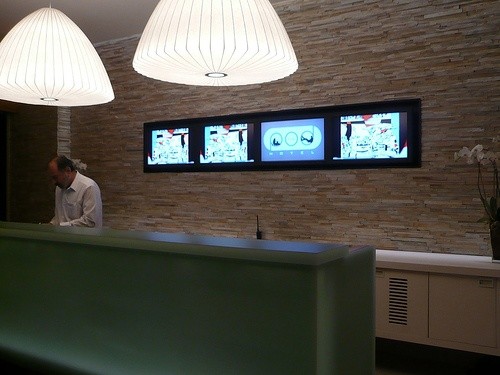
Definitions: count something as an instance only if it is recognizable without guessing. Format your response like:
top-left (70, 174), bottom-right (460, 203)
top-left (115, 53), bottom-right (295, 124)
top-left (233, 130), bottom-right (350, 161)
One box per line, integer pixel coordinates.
top-left (488, 218), bottom-right (500, 263)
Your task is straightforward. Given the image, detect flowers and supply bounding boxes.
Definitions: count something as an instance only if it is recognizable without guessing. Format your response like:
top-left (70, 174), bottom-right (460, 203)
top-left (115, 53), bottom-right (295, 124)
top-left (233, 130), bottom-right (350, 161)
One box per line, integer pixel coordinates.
top-left (454, 135), bottom-right (500, 226)
top-left (70, 159), bottom-right (88, 171)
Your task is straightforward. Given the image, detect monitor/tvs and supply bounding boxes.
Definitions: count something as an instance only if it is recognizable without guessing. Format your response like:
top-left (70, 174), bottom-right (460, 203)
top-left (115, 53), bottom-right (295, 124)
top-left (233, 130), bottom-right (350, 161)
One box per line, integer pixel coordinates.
top-left (330, 107), bottom-right (414, 160)
top-left (147, 125), bottom-right (194, 164)
top-left (196, 123), bottom-right (254, 164)
top-left (256, 115), bottom-right (329, 163)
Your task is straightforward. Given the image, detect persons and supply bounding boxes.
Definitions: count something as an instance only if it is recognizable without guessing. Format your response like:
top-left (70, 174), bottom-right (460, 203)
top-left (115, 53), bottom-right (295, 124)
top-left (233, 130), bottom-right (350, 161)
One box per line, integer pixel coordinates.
top-left (48, 155), bottom-right (102, 228)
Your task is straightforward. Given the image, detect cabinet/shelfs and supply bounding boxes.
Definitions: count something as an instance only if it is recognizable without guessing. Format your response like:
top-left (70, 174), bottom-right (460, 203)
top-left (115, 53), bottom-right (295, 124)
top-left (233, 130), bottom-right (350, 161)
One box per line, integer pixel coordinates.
top-left (428, 272), bottom-right (500, 357)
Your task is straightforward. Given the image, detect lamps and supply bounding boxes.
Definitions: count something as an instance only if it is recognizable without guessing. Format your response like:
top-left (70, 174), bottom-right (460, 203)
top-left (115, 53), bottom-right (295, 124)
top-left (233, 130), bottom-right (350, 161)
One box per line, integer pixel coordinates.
top-left (0, 0), bottom-right (115, 107)
top-left (132, 0), bottom-right (299, 86)
